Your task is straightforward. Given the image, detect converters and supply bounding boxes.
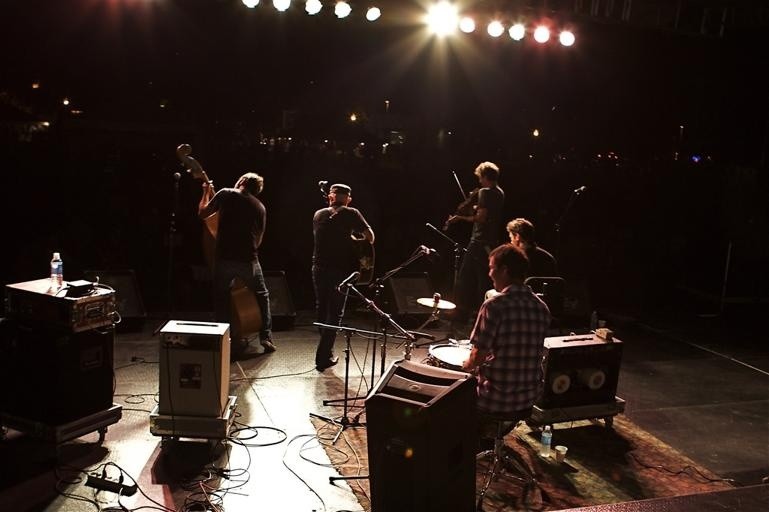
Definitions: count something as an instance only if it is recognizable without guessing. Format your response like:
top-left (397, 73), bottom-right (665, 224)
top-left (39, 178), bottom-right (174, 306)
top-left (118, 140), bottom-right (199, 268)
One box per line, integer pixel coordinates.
top-left (66, 279), bottom-right (93, 297)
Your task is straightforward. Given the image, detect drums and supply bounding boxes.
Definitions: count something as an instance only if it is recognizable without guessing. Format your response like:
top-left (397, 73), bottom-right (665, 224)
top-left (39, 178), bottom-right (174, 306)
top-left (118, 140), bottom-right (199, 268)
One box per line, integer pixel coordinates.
top-left (427, 344), bottom-right (471, 371)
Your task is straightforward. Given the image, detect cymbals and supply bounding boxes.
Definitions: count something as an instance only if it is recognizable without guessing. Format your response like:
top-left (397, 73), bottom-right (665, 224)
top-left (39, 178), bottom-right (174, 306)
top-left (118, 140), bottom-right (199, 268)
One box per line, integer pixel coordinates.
top-left (416, 298), bottom-right (456, 310)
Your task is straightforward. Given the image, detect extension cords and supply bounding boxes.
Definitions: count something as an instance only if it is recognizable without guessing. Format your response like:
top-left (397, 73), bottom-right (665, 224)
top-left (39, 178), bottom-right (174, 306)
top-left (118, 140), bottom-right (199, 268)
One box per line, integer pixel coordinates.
top-left (87, 471), bottom-right (137, 496)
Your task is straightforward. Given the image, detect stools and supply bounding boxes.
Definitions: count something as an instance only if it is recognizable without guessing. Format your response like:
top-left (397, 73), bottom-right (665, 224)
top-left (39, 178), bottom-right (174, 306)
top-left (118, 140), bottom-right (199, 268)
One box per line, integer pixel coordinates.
top-left (475, 408), bottom-right (535, 511)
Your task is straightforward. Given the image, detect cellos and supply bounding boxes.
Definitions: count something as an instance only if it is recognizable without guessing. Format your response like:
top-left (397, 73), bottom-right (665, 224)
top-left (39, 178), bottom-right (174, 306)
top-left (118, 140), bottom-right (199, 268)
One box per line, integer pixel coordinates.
top-left (176, 143), bottom-right (264, 335)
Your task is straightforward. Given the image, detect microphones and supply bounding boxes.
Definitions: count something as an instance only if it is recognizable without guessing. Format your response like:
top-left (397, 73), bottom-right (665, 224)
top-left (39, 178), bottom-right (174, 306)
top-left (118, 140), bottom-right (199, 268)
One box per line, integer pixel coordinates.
top-left (407, 330), bottom-right (436, 341)
top-left (421, 244), bottom-right (441, 264)
top-left (338, 270), bottom-right (361, 290)
top-left (173, 172), bottom-right (182, 191)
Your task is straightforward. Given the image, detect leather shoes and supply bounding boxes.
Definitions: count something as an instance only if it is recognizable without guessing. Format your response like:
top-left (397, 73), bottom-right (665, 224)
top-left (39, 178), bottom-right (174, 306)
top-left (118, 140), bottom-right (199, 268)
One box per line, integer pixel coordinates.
top-left (315, 355), bottom-right (340, 369)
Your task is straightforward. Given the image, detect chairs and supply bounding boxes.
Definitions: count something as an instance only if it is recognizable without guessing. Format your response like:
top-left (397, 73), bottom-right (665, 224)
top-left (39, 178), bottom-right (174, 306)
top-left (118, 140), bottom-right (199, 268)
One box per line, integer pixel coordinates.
top-left (524, 275), bottom-right (566, 318)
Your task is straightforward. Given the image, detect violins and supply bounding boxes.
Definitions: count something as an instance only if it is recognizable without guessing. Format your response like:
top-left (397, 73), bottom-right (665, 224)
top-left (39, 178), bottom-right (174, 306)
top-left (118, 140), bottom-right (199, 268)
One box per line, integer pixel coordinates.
top-left (443, 188), bottom-right (479, 231)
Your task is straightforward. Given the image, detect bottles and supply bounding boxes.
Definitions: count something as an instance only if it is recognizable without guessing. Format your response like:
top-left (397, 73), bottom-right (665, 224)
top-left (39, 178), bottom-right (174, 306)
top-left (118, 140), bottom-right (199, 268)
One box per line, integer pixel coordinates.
top-left (591, 311), bottom-right (597, 332)
top-left (540, 425), bottom-right (553, 458)
top-left (50, 252), bottom-right (63, 291)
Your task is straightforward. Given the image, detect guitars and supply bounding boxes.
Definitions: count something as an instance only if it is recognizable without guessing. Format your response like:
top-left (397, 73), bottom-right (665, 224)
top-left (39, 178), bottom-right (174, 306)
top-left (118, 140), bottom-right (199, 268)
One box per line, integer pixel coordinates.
top-left (318, 181), bottom-right (375, 285)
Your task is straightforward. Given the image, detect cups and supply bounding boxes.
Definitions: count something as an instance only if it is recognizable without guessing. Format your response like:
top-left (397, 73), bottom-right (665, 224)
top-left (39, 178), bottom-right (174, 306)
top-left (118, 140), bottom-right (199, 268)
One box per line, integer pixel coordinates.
top-left (555, 445), bottom-right (568, 463)
top-left (598, 320), bottom-right (606, 327)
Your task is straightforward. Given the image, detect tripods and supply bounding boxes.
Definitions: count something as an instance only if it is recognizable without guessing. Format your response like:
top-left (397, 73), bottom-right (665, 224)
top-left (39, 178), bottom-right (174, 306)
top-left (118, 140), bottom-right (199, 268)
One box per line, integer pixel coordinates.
top-left (310, 322), bottom-right (404, 444)
top-left (322, 251), bottom-right (423, 406)
top-left (329, 285), bottom-right (415, 482)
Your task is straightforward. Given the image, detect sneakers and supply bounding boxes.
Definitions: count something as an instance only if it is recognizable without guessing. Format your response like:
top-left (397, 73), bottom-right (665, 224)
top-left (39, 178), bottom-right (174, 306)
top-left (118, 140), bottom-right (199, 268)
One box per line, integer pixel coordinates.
top-left (261, 340), bottom-right (277, 352)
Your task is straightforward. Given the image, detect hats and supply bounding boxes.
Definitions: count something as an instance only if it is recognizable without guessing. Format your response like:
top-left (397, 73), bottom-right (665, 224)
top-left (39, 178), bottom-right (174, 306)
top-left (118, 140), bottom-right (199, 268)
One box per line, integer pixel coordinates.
top-left (330, 183), bottom-right (352, 196)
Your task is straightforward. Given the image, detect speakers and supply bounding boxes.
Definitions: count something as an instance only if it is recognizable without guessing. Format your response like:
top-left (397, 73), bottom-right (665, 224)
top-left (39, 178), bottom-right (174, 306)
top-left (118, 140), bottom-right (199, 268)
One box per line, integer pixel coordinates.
top-left (364, 357), bottom-right (477, 512)
top-left (534, 334), bottom-right (624, 409)
top-left (388, 272), bottom-right (441, 329)
top-left (262, 270), bottom-right (297, 327)
top-left (0, 318), bottom-right (117, 425)
top-left (85, 269), bottom-right (147, 333)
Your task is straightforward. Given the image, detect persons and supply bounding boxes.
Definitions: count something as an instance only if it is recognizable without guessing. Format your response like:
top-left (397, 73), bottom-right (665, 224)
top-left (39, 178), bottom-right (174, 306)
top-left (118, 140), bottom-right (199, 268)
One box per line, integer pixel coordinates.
top-left (501, 214), bottom-right (568, 286)
top-left (308, 183), bottom-right (377, 369)
top-left (457, 242), bottom-right (555, 420)
top-left (441, 158), bottom-right (510, 337)
top-left (197, 170), bottom-right (279, 362)
